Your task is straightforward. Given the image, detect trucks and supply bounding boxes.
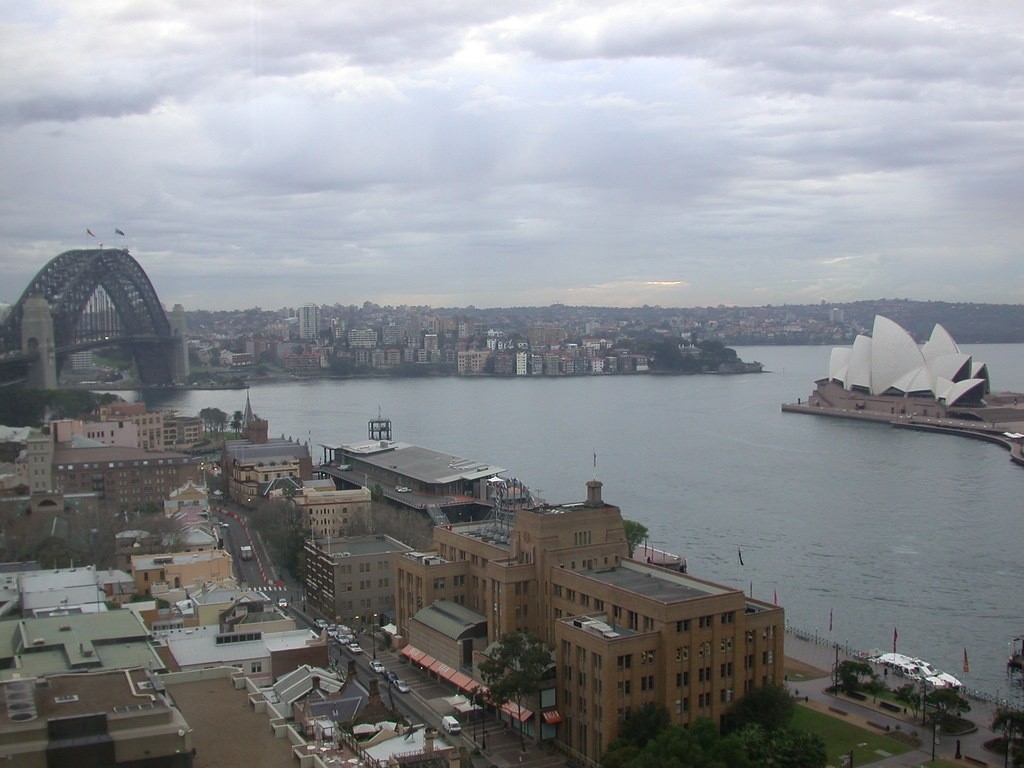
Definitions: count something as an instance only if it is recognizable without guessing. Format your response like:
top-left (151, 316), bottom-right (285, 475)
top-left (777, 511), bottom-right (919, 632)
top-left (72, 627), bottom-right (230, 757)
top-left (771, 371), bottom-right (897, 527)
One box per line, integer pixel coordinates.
top-left (240, 546), bottom-right (252, 561)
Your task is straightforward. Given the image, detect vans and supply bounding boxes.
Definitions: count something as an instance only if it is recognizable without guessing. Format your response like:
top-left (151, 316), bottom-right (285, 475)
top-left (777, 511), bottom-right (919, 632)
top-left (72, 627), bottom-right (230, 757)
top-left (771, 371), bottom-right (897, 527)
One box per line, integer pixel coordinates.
top-left (443, 716), bottom-right (462, 735)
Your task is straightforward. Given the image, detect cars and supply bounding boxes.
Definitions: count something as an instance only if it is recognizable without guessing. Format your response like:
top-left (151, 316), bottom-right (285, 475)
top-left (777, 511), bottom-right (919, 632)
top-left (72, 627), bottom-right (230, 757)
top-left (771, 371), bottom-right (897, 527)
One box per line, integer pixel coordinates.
top-left (279, 598), bottom-right (288, 608)
top-left (317, 619), bottom-right (411, 694)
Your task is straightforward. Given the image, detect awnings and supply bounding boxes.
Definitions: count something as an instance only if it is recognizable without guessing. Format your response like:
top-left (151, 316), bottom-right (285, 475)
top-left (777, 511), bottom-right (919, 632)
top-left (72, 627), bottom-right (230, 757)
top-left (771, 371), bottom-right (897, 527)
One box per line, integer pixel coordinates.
top-left (402, 644), bottom-right (562, 724)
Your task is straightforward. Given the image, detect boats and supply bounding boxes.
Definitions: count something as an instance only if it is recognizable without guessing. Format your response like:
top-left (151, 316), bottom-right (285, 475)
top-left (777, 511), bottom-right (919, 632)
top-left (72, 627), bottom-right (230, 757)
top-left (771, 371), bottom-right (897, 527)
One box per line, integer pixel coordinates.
top-left (874, 651), bottom-right (963, 691)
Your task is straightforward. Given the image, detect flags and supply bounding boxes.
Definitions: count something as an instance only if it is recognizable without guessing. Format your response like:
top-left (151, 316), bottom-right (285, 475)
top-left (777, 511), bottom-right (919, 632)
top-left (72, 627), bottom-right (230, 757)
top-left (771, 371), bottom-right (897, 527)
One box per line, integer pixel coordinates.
top-left (829, 608), bottom-right (834, 631)
top-left (963, 649), bottom-right (969, 672)
top-left (737, 546), bottom-right (743, 566)
top-left (892, 628), bottom-right (898, 652)
top-left (87, 229), bottom-right (95, 237)
top-left (773, 590), bottom-right (778, 605)
top-left (114, 228), bottom-right (125, 236)
